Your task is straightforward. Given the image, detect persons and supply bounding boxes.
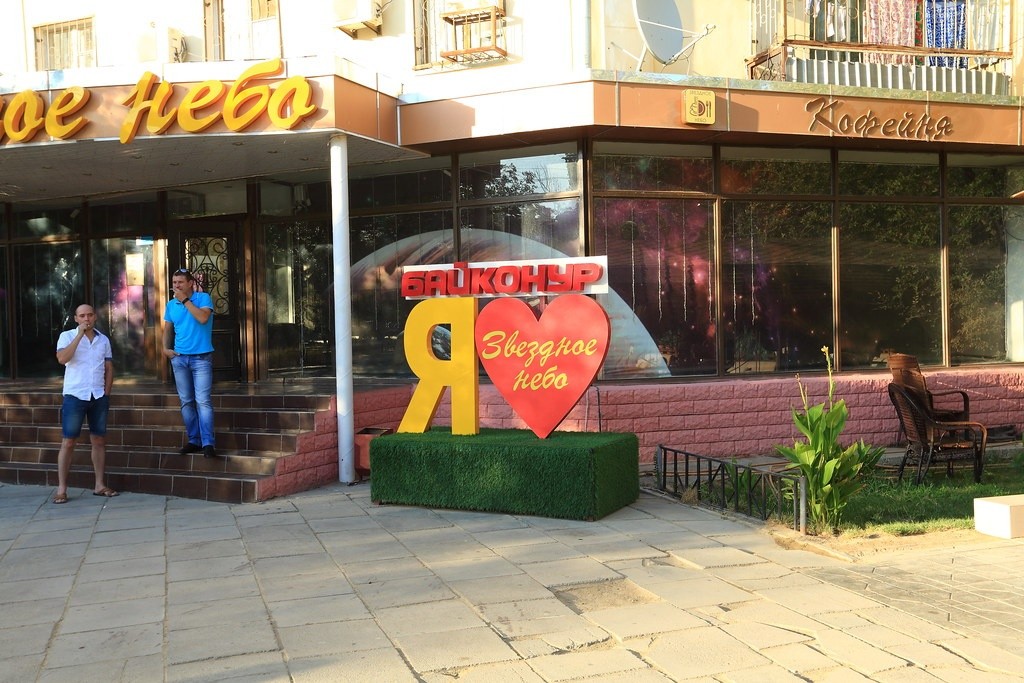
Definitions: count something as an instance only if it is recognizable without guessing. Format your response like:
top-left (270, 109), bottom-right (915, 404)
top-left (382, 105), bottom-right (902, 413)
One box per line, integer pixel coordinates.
top-left (162, 269), bottom-right (217, 460)
top-left (54, 304), bottom-right (119, 503)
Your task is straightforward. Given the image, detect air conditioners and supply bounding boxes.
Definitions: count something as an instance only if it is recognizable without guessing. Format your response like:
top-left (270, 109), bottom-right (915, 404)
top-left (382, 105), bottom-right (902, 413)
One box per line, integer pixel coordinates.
top-left (137, 27), bottom-right (182, 64)
top-left (331, 0), bottom-right (383, 30)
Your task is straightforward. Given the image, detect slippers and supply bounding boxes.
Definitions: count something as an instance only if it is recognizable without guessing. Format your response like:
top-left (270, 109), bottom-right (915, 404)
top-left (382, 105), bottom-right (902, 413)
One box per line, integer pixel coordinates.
top-left (93, 487), bottom-right (120, 496)
top-left (53, 491), bottom-right (67, 504)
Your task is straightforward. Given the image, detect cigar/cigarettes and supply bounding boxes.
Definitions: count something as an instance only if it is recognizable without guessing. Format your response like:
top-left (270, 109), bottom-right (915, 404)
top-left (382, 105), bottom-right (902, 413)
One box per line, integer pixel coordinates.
top-left (170, 288), bottom-right (176, 291)
top-left (87, 324), bottom-right (90, 327)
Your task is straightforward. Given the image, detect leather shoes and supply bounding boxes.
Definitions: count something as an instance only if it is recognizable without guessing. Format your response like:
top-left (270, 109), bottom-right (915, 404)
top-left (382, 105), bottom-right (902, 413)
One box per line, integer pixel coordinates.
top-left (179, 443), bottom-right (202, 453)
top-left (203, 445), bottom-right (215, 458)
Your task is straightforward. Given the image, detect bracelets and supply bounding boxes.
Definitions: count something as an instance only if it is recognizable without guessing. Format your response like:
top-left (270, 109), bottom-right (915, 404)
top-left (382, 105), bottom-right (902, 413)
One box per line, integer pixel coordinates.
top-left (182, 297), bottom-right (189, 304)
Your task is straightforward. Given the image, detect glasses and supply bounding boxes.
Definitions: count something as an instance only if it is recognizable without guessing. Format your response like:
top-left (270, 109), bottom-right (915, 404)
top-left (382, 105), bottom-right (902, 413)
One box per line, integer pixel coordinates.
top-left (174, 269), bottom-right (189, 274)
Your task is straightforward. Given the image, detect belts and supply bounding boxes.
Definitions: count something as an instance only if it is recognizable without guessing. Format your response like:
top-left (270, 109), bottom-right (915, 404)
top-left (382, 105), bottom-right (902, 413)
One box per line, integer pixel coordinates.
top-left (181, 352), bottom-right (209, 356)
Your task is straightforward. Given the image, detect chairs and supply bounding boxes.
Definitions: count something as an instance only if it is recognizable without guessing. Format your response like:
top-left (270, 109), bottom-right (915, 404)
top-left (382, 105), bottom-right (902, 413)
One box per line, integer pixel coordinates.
top-left (887, 353), bottom-right (970, 450)
top-left (887, 383), bottom-right (988, 486)
